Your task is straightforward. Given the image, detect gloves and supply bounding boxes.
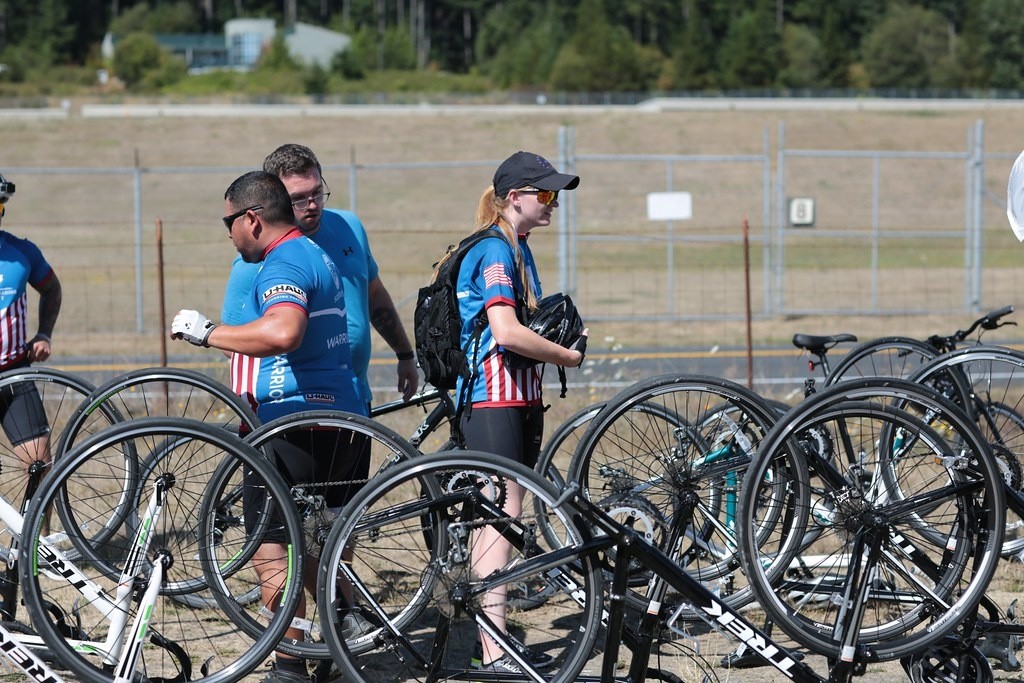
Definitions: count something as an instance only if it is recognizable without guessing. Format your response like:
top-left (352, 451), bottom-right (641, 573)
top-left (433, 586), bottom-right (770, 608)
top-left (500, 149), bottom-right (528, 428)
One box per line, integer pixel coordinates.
top-left (170, 309), bottom-right (217, 348)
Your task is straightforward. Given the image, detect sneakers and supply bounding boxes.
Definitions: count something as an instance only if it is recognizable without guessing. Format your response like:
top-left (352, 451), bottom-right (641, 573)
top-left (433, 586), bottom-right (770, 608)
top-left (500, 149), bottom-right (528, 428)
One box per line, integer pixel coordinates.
top-left (309, 608), bottom-right (375, 683)
top-left (475, 655), bottom-right (528, 675)
top-left (257, 659), bottom-right (314, 683)
top-left (471, 642), bottom-right (556, 673)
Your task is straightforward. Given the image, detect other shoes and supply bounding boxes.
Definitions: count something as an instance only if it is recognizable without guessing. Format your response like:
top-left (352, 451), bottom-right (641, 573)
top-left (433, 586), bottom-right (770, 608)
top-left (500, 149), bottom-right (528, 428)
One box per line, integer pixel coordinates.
top-left (39, 565), bottom-right (64, 580)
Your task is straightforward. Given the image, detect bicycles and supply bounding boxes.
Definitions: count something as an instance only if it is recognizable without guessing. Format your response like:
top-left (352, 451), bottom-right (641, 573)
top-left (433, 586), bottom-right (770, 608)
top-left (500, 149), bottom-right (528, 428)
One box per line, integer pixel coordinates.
top-left (1, 304), bottom-right (1024, 683)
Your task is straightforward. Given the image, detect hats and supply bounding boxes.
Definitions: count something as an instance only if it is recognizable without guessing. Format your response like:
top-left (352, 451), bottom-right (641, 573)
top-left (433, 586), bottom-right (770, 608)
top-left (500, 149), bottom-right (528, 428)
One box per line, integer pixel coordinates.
top-left (493, 151), bottom-right (580, 191)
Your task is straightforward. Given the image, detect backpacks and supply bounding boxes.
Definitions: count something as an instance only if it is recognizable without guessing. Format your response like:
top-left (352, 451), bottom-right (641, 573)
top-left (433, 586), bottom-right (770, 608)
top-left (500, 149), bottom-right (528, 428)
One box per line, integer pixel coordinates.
top-left (413, 229), bottom-right (511, 390)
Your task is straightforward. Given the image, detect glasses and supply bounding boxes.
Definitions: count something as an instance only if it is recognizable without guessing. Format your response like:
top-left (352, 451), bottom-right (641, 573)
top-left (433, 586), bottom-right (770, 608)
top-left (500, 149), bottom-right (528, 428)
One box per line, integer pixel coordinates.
top-left (519, 190), bottom-right (558, 205)
top-left (291, 177), bottom-right (331, 211)
top-left (223, 205), bottom-right (264, 234)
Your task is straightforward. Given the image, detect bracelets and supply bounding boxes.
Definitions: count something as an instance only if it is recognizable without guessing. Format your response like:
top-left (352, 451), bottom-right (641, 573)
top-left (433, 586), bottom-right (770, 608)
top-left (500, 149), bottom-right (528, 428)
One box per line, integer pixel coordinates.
top-left (396, 351), bottom-right (414, 361)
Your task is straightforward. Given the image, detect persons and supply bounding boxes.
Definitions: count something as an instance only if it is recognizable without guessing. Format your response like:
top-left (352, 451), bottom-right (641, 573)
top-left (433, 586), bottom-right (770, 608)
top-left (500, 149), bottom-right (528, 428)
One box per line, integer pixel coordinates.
top-left (171, 142), bottom-right (418, 683)
top-left (0, 175), bottom-right (67, 582)
top-left (437, 152), bottom-right (589, 675)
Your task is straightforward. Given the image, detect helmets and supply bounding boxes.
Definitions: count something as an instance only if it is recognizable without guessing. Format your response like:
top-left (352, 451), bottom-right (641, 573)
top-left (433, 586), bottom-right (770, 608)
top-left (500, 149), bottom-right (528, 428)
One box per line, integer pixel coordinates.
top-left (0, 174), bottom-right (16, 202)
top-left (502, 292), bottom-right (584, 369)
